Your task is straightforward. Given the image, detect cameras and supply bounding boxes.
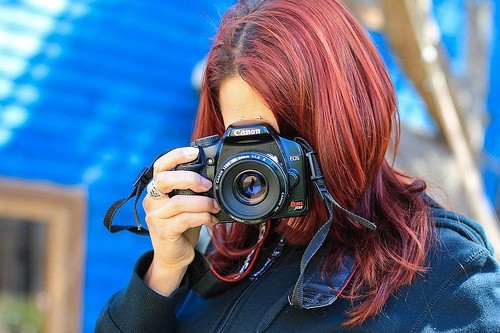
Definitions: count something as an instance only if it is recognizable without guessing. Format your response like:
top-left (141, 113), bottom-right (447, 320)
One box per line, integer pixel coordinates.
top-left (175, 120), bottom-right (312, 227)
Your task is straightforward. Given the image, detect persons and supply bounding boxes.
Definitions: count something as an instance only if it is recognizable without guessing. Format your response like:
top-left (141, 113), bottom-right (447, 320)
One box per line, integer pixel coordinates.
top-left (92, 0), bottom-right (500, 333)
